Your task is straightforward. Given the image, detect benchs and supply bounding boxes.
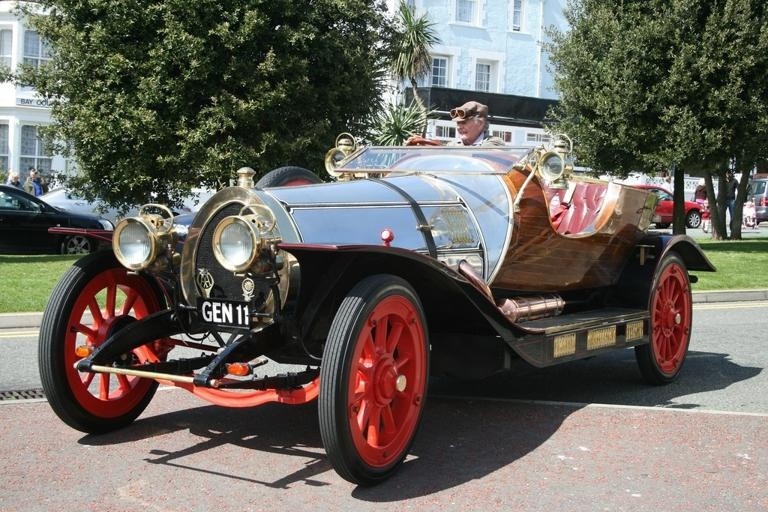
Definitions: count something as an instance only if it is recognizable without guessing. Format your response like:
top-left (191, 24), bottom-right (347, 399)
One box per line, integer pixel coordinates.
top-left (540, 177), bottom-right (608, 235)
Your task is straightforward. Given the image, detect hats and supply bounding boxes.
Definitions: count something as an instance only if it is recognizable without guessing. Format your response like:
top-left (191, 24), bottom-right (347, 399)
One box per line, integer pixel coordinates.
top-left (452, 101), bottom-right (488, 122)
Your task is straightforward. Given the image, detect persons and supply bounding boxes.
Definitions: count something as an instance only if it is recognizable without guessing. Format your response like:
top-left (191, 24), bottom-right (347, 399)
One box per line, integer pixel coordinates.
top-left (23, 167), bottom-right (44, 195)
top-left (6, 173), bottom-right (24, 191)
top-left (699, 170), bottom-right (756, 234)
top-left (404, 101), bottom-right (510, 153)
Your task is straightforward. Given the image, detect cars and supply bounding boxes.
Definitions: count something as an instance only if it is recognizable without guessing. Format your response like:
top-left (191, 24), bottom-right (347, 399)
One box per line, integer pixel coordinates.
top-left (36, 134), bottom-right (717, 488)
top-left (746, 179), bottom-right (768, 223)
top-left (633, 183), bottom-right (703, 230)
top-left (0, 185), bottom-right (191, 257)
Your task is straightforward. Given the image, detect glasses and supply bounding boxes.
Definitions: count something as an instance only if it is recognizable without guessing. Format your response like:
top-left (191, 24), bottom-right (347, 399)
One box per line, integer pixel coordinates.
top-left (450, 109), bottom-right (480, 119)
